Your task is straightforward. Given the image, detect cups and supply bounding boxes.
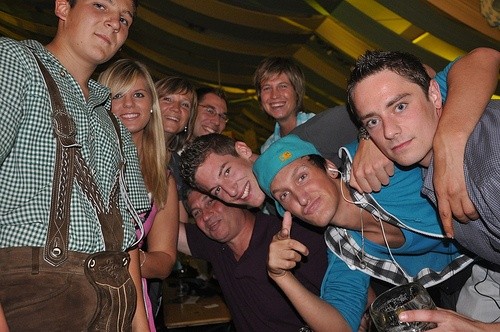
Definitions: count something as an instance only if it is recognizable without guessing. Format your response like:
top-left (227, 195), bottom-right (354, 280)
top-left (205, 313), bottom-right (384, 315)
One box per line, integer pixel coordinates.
top-left (369, 282), bottom-right (439, 332)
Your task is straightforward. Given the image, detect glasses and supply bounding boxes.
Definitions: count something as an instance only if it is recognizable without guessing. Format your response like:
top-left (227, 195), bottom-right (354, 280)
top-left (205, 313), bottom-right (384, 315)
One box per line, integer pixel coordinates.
top-left (198, 103), bottom-right (231, 123)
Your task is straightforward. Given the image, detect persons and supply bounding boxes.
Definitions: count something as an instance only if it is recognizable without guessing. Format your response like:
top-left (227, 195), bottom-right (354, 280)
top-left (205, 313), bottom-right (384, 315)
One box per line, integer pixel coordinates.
top-left (0, 0), bottom-right (152, 332)
top-left (98, 47), bottom-right (500, 332)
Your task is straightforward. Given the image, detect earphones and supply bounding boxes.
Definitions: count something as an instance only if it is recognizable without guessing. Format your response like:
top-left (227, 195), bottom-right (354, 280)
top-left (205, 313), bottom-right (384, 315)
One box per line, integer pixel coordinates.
top-left (329, 168), bottom-right (334, 171)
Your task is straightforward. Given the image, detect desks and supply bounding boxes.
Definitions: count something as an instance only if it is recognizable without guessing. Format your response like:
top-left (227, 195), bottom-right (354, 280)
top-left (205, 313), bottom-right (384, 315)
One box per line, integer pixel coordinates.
top-left (164, 296), bottom-right (231, 332)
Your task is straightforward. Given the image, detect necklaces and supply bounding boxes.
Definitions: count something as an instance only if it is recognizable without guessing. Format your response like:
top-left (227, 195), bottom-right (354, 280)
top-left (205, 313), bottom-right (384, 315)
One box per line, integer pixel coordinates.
top-left (344, 207), bottom-right (368, 270)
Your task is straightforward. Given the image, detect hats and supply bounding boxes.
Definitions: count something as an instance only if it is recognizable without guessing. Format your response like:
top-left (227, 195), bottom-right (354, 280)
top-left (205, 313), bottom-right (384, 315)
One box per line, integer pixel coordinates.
top-left (252, 134), bottom-right (324, 219)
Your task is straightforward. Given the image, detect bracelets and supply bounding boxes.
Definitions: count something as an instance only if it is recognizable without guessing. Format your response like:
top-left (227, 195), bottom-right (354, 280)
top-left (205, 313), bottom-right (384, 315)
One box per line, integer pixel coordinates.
top-left (138, 249), bottom-right (146, 267)
